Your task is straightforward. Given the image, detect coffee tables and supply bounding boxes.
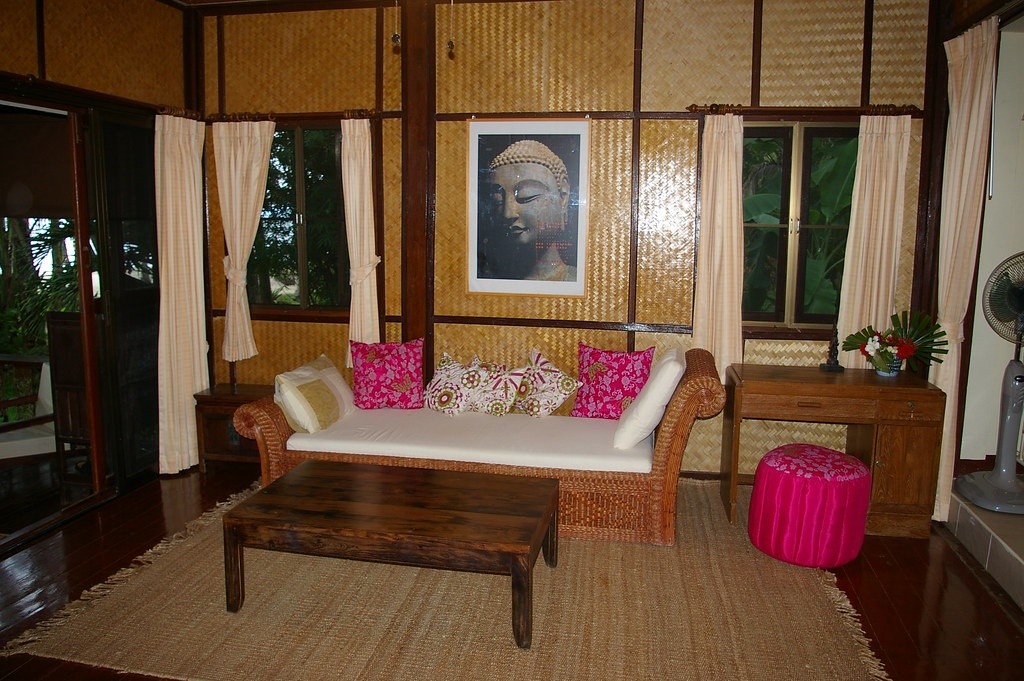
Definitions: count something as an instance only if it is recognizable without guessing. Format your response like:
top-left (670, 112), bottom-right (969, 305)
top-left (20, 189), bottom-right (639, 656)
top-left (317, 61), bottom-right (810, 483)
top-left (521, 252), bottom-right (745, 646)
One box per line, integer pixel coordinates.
top-left (223, 459), bottom-right (560, 648)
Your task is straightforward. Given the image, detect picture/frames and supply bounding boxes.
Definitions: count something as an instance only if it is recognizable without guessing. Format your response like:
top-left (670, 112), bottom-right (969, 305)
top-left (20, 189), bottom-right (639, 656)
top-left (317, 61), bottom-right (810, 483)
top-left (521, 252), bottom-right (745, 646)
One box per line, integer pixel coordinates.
top-left (465, 117), bottom-right (593, 298)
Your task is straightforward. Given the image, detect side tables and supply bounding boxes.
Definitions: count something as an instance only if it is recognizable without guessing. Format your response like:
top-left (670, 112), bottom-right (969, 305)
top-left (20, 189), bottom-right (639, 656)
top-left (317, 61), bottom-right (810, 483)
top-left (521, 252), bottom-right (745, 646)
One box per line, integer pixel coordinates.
top-left (193, 382), bottom-right (275, 473)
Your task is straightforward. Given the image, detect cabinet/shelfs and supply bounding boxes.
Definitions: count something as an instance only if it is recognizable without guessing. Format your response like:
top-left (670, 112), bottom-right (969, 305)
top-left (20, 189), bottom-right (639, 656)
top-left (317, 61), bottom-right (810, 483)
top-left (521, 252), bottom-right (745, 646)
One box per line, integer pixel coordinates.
top-left (720, 362), bottom-right (947, 538)
top-left (47, 311), bottom-right (112, 481)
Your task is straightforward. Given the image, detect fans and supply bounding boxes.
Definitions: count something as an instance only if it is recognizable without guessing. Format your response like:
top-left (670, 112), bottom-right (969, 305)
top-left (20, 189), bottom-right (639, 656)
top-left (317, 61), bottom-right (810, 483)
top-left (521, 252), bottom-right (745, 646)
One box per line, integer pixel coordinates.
top-left (954, 250), bottom-right (1024, 514)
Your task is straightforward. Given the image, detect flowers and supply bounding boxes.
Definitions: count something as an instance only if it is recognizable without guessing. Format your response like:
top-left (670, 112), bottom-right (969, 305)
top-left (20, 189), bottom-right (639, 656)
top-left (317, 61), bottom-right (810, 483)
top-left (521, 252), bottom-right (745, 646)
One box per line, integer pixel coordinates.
top-left (859, 331), bottom-right (917, 358)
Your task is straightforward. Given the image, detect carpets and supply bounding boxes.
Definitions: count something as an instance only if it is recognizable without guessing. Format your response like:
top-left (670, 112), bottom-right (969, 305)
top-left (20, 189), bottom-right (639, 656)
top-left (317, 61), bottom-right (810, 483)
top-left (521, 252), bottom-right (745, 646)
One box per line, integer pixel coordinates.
top-left (0, 476), bottom-right (893, 681)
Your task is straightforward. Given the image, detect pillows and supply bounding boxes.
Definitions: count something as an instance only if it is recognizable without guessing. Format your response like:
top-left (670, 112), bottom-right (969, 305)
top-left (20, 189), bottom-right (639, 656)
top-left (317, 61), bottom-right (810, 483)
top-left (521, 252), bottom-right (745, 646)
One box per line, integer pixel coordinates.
top-left (423, 351), bottom-right (489, 417)
top-left (571, 338), bottom-right (656, 420)
top-left (274, 351), bottom-right (359, 434)
top-left (465, 354), bottom-right (529, 417)
top-left (507, 346), bottom-right (584, 418)
top-left (614, 347), bottom-right (688, 447)
top-left (350, 335), bottom-right (426, 408)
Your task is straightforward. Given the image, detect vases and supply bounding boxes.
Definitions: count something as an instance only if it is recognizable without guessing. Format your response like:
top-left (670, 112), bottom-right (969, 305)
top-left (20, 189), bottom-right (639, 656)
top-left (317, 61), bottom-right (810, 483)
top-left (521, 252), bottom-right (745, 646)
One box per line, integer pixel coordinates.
top-left (875, 354), bottom-right (902, 376)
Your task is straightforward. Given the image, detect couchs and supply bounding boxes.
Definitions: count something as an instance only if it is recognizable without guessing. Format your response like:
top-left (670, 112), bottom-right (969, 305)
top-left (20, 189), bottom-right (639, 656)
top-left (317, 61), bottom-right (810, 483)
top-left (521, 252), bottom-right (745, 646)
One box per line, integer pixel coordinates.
top-left (232, 346), bottom-right (727, 546)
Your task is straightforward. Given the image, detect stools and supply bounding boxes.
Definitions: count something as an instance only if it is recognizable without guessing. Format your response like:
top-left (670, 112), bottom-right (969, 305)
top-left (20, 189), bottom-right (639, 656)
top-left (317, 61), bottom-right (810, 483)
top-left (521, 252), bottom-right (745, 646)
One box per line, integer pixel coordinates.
top-left (748, 443), bottom-right (873, 568)
